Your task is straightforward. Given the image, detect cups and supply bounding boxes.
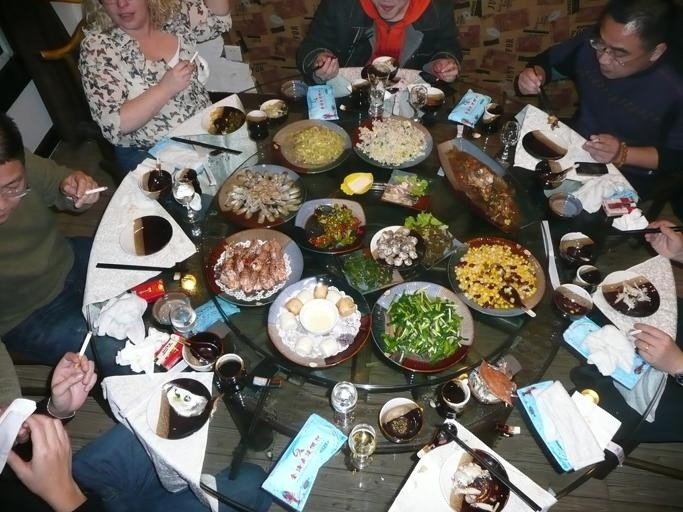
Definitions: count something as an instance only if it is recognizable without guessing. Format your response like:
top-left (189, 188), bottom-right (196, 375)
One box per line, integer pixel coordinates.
top-left (442, 378), bottom-right (471, 414)
top-left (538, 158), bottom-right (569, 186)
top-left (212, 354), bottom-right (244, 381)
top-left (554, 284), bottom-right (593, 322)
top-left (558, 232), bottom-right (600, 266)
top-left (573, 261), bottom-right (601, 287)
top-left (279, 76), bottom-right (312, 100)
top-left (258, 99), bottom-right (292, 119)
top-left (173, 168), bottom-right (203, 193)
top-left (368, 54), bottom-right (398, 76)
top-left (181, 331), bottom-right (221, 372)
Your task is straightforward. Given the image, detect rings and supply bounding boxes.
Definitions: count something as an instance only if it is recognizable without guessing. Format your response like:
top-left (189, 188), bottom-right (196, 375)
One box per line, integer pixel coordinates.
top-left (644, 344), bottom-right (649, 352)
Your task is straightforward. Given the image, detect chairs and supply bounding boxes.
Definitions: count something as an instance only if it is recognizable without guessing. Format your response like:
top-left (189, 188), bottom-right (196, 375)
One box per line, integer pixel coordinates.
top-left (559, 102), bottom-right (677, 219)
top-left (40, 16), bottom-right (116, 177)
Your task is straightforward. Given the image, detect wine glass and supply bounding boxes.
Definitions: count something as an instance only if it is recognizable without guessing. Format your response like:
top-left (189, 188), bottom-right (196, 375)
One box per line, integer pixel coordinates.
top-left (346, 425), bottom-right (380, 472)
top-left (408, 84), bottom-right (430, 118)
top-left (500, 117), bottom-right (520, 166)
top-left (168, 301), bottom-right (191, 338)
top-left (331, 381), bottom-right (359, 426)
top-left (168, 175), bottom-right (203, 225)
top-left (246, 107), bottom-right (269, 145)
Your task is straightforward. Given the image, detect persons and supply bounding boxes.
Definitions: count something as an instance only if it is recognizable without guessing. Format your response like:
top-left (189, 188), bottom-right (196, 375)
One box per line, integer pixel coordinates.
top-left (77, 0), bottom-right (232, 171)
top-left (0, 113), bottom-right (127, 365)
top-left (295, 0), bottom-right (463, 85)
top-left (0, 352), bottom-right (271, 512)
top-left (628, 221), bottom-right (683, 383)
top-left (514, 1), bottom-right (683, 200)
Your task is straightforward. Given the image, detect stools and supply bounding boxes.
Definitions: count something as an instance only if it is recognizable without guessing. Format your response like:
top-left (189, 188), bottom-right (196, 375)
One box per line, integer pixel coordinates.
top-left (595, 430), bottom-right (682, 489)
top-left (6, 343), bottom-right (61, 399)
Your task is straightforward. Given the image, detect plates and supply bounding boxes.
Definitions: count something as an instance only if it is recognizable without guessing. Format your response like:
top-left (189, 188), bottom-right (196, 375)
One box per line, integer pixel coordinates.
top-left (547, 192), bottom-right (586, 221)
top-left (207, 228), bottom-right (302, 311)
top-left (522, 129), bottom-right (573, 163)
top-left (203, 104), bottom-right (246, 136)
top-left (152, 292), bottom-right (191, 324)
top-left (296, 197), bottom-right (368, 256)
top-left (370, 285), bottom-right (477, 373)
top-left (143, 380), bottom-right (213, 440)
top-left (270, 118), bottom-right (354, 172)
top-left (437, 448), bottom-right (513, 508)
top-left (377, 396), bottom-right (427, 440)
top-left (448, 238), bottom-right (546, 318)
top-left (116, 214), bottom-right (173, 256)
top-left (468, 364), bottom-right (515, 408)
top-left (327, 216), bottom-right (462, 297)
top-left (267, 276), bottom-right (368, 368)
top-left (217, 162), bottom-right (304, 227)
top-left (482, 101), bottom-right (499, 124)
top-left (353, 120), bottom-right (435, 161)
top-left (435, 136), bottom-right (539, 230)
top-left (600, 270), bottom-right (661, 319)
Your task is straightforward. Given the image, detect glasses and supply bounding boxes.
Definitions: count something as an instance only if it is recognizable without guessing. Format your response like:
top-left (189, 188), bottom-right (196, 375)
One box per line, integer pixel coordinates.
top-left (589, 39), bottom-right (651, 69)
top-left (0, 184), bottom-right (32, 199)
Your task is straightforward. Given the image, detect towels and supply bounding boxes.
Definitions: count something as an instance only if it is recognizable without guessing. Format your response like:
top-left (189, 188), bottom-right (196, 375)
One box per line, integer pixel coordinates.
top-left (97, 370), bottom-right (218, 510)
top-left (336, 65), bottom-right (428, 90)
top-left (591, 252), bottom-right (678, 425)
top-left (81, 168), bottom-right (201, 330)
top-left (166, 91), bottom-right (263, 199)
top-left (509, 103), bottom-right (639, 215)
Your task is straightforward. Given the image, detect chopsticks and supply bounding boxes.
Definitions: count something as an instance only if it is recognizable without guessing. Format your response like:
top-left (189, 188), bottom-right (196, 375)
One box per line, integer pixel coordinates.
top-left (94, 257), bottom-right (189, 277)
top-left (433, 418), bottom-right (546, 512)
top-left (228, 377), bottom-right (273, 481)
top-left (170, 135), bottom-right (241, 157)
top-left (605, 213), bottom-right (683, 245)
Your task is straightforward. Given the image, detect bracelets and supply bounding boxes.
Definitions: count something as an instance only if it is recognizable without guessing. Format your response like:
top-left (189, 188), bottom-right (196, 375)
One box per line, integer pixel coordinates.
top-left (616, 141), bottom-right (628, 169)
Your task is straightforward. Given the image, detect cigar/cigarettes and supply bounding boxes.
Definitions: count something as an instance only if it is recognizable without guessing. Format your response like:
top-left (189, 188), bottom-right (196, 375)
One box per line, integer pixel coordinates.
top-left (78, 331), bottom-right (94, 362)
top-left (628, 330), bottom-right (642, 335)
top-left (593, 138), bottom-right (600, 143)
top-left (83, 186), bottom-right (107, 195)
top-left (190, 51), bottom-right (199, 65)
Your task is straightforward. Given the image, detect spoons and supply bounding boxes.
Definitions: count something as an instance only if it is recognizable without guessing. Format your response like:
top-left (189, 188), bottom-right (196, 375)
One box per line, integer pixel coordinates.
top-left (416, 422), bottom-right (457, 460)
